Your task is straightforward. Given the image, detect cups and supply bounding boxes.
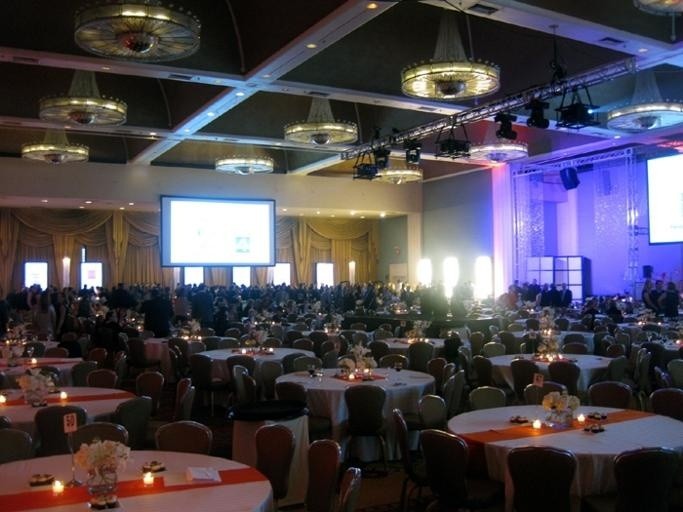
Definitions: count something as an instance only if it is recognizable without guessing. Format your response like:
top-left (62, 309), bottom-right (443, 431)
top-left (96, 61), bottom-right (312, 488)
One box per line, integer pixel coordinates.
top-left (142, 473), bottom-right (155, 490)
top-left (52, 480), bottom-right (63, 497)
top-left (30, 335), bottom-right (38, 342)
top-left (1, 357), bottom-right (68, 403)
top-left (662, 316), bottom-right (678, 324)
top-left (584, 411), bottom-right (608, 433)
top-left (306, 363), bottom-right (323, 378)
top-left (394, 361), bottom-right (402, 372)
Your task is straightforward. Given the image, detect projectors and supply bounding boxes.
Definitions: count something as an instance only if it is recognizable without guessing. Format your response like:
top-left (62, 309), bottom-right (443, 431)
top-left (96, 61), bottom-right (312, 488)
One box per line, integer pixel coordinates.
top-left (357, 164), bottom-right (377, 177)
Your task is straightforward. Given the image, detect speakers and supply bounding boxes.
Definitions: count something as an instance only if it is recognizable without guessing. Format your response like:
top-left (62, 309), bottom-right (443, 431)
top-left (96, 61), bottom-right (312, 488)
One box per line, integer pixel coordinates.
top-left (559, 167), bottom-right (580, 189)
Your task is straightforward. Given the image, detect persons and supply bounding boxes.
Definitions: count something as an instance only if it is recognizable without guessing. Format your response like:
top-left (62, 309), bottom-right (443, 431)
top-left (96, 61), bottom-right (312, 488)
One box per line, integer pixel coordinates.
top-left (1, 271), bottom-right (681, 365)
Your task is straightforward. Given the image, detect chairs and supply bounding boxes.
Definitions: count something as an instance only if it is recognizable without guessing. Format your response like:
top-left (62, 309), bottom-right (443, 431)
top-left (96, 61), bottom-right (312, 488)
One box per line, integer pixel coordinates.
top-left (0, 300), bottom-right (683, 512)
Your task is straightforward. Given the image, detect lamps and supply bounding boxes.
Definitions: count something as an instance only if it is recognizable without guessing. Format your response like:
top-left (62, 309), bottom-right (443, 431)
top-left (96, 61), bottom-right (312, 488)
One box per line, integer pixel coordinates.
top-left (74, 1), bottom-right (200, 63)
top-left (399, 60), bottom-right (504, 100)
top-left (282, 121), bottom-right (356, 146)
top-left (376, 169), bottom-right (422, 183)
top-left (526, 109), bottom-right (549, 129)
top-left (467, 142), bottom-right (528, 163)
top-left (372, 149), bottom-right (389, 168)
top-left (607, 103), bottom-right (683, 133)
top-left (38, 98), bottom-right (128, 130)
top-left (405, 149), bottom-right (420, 165)
top-left (216, 155), bottom-right (277, 175)
top-left (497, 120), bottom-right (517, 140)
top-left (20, 146), bottom-right (88, 167)
top-left (559, 167), bottom-right (582, 191)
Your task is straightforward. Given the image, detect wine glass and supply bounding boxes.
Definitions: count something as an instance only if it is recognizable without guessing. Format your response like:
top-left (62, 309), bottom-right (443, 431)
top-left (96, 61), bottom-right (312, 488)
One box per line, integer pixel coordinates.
top-left (46, 329), bottom-right (52, 345)
top-left (27, 346), bottom-right (34, 359)
top-left (646, 331), bottom-right (652, 342)
